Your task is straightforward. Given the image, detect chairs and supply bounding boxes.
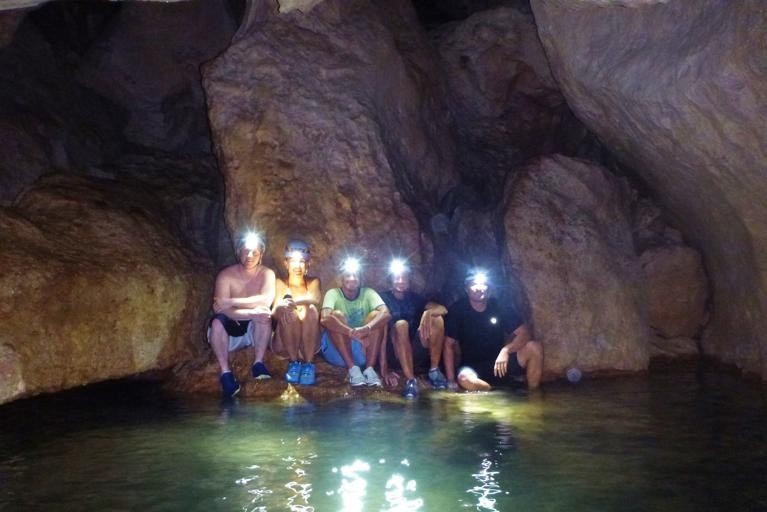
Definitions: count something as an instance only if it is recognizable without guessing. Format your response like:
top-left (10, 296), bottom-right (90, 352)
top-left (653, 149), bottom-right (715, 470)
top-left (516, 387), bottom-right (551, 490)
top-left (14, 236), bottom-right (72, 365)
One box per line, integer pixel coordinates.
top-left (252, 361), bottom-right (272, 380)
top-left (347, 366), bottom-right (382, 388)
top-left (284, 359), bottom-right (315, 385)
top-left (402, 378), bottom-right (417, 397)
top-left (425, 367), bottom-right (448, 390)
top-left (220, 371), bottom-right (241, 397)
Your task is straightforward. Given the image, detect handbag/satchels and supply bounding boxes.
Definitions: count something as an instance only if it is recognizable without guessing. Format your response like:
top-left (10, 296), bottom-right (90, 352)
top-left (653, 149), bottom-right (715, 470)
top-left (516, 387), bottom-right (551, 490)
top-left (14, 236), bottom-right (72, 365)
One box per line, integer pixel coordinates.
top-left (365, 323), bottom-right (372, 334)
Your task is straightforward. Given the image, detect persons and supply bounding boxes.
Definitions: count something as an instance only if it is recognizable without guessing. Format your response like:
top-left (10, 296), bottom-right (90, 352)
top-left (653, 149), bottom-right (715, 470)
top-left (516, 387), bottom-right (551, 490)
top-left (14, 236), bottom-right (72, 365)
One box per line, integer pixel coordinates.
top-left (275, 241), bottom-right (325, 385)
top-left (374, 256), bottom-right (448, 398)
top-left (442, 266), bottom-right (544, 390)
top-left (204, 233), bottom-right (275, 397)
top-left (319, 255), bottom-right (391, 388)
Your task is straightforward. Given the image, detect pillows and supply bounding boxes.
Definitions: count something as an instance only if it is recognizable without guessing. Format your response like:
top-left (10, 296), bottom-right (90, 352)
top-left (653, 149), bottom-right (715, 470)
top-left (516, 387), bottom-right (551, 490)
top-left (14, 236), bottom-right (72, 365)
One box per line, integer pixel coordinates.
top-left (336, 258), bottom-right (362, 272)
top-left (235, 232), bottom-right (266, 257)
top-left (386, 257), bottom-right (412, 283)
top-left (285, 240), bottom-right (311, 260)
top-left (464, 266), bottom-right (496, 297)
